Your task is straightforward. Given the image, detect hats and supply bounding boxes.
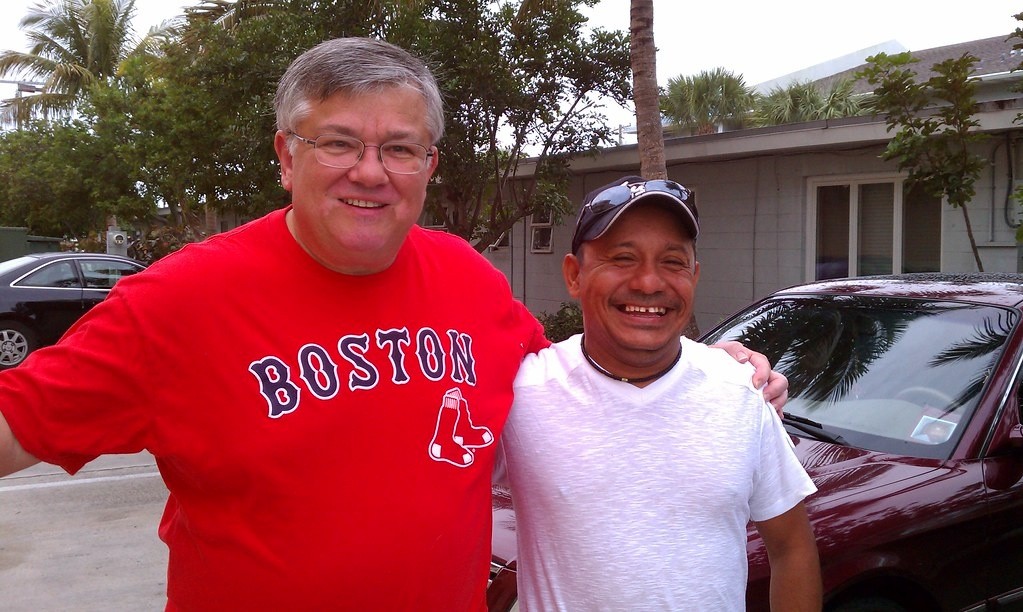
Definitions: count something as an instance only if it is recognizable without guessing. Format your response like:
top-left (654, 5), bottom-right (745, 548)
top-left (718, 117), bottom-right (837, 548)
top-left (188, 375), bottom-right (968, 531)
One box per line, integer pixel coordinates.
top-left (575, 176), bottom-right (700, 242)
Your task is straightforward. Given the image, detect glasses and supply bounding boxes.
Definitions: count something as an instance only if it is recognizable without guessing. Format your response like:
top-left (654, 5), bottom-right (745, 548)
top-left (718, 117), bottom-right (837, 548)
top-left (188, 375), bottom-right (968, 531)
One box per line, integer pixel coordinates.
top-left (287, 128), bottom-right (434, 176)
top-left (571, 178), bottom-right (696, 254)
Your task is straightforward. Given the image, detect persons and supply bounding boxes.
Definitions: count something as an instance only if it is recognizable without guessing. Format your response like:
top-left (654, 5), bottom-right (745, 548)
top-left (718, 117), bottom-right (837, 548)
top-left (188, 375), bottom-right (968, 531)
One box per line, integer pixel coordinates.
top-left (0, 36), bottom-right (823, 612)
top-left (912, 421), bottom-right (954, 444)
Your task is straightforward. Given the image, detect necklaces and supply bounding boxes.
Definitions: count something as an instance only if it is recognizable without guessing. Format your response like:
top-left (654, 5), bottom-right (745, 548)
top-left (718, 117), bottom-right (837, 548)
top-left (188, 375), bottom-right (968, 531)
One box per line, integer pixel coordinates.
top-left (581, 332), bottom-right (682, 383)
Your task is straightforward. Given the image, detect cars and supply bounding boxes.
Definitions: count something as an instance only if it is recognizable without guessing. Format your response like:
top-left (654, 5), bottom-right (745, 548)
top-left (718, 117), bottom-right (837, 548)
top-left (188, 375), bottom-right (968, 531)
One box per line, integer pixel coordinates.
top-left (0, 252), bottom-right (154, 369)
top-left (476, 273), bottom-right (1023, 612)
